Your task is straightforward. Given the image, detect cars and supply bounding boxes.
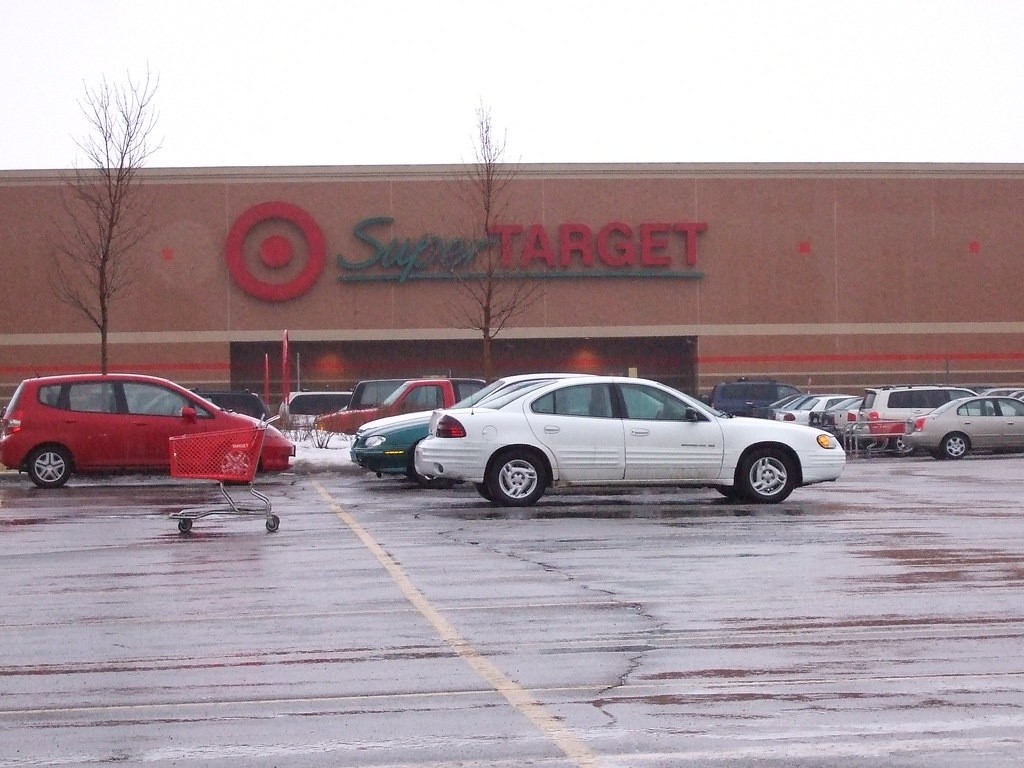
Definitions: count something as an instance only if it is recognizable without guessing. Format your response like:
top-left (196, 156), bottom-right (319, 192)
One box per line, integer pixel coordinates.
top-left (767, 394), bottom-right (864, 450)
top-left (903, 396), bottom-right (1024, 460)
top-left (0, 373), bottom-right (296, 489)
top-left (313, 380), bottom-right (452, 435)
top-left (980, 387), bottom-right (1024, 404)
top-left (350, 373), bottom-right (664, 488)
top-left (415, 376), bottom-right (848, 508)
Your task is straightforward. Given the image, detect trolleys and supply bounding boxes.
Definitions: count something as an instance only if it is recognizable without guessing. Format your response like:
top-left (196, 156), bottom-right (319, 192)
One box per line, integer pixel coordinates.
top-left (168, 412), bottom-right (281, 533)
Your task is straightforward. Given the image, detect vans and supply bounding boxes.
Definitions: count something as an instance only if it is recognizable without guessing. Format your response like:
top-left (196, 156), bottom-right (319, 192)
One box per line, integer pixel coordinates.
top-left (707, 379), bottom-right (801, 421)
top-left (277, 391), bottom-right (355, 426)
top-left (344, 377), bottom-right (489, 412)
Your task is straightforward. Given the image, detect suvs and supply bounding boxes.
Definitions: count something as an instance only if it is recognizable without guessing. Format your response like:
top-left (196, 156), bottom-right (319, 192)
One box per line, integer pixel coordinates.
top-left (851, 384), bottom-right (1016, 458)
top-left (188, 387), bottom-right (271, 420)
